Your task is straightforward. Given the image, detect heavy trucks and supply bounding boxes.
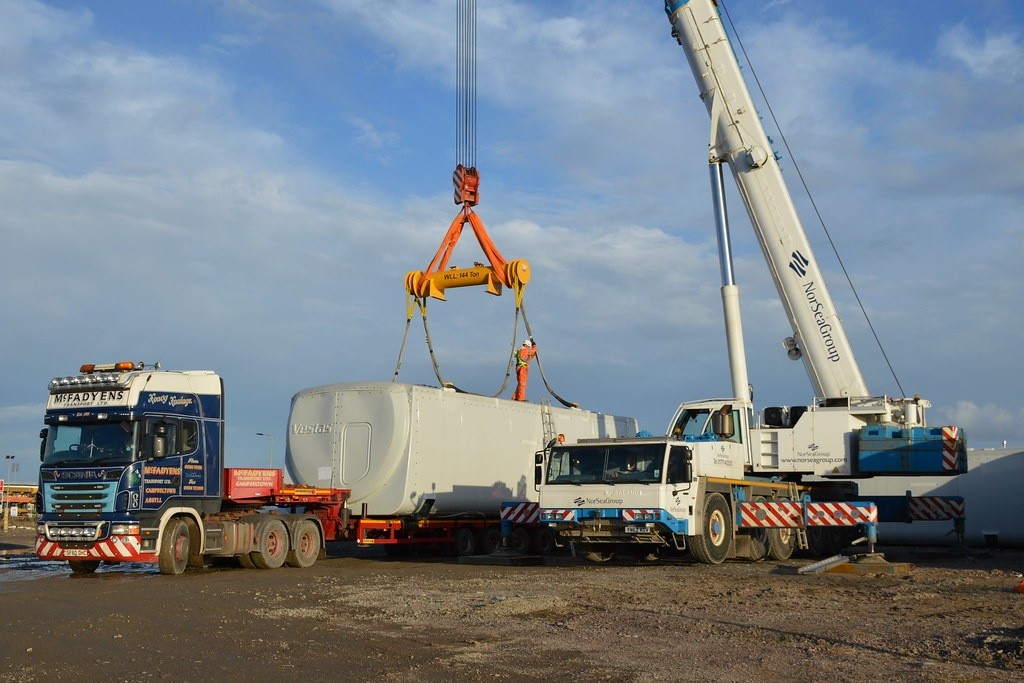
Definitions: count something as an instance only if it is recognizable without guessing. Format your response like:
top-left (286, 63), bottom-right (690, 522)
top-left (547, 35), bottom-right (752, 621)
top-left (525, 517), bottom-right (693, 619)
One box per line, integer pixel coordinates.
top-left (34, 361), bottom-right (569, 576)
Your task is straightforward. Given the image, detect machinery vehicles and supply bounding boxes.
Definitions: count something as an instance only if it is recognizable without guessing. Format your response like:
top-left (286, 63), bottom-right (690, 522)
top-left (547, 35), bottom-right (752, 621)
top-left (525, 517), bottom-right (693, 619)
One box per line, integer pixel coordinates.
top-left (404, 1), bottom-right (968, 480)
top-left (534, 1), bottom-right (966, 566)
